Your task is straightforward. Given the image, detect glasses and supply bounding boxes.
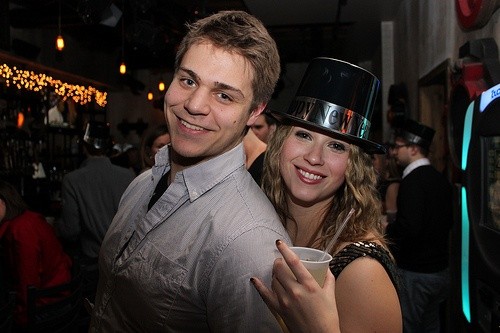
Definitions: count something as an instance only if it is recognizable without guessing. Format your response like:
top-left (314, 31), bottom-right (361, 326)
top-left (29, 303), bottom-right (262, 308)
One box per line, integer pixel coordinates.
top-left (390, 142), bottom-right (415, 150)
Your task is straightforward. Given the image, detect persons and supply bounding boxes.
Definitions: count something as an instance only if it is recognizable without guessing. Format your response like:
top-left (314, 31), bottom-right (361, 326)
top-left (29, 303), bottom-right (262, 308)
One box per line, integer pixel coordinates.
top-left (250, 113), bottom-right (276, 142)
top-left (392, 118), bottom-right (460, 333)
top-left (1, 114), bottom-right (172, 332)
top-left (243, 128), bottom-right (269, 185)
top-left (371, 142), bottom-right (401, 225)
top-left (89, 8), bottom-right (297, 333)
top-left (249, 56), bottom-right (404, 333)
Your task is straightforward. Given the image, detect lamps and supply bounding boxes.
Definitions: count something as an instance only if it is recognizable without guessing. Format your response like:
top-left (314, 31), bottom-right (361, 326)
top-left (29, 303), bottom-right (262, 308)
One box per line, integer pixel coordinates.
top-left (119, 0), bottom-right (127, 74)
top-left (56, 0), bottom-right (65, 52)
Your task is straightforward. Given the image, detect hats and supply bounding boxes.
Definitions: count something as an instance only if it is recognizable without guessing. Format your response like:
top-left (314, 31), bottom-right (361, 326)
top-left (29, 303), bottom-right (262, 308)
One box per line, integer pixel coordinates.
top-left (265, 58), bottom-right (387, 154)
top-left (81, 121), bottom-right (114, 149)
top-left (394, 118), bottom-right (435, 152)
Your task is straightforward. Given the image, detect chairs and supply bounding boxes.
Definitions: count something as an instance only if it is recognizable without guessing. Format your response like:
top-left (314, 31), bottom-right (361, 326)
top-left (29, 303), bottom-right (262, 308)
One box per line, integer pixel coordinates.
top-left (73, 256), bottom-right (99, 333)
top-left (27, 269), bottom-right (88, 333)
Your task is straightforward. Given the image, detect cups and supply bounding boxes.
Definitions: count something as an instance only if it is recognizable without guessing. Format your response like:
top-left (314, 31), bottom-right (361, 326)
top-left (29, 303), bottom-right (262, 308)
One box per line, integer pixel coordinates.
top-left (282, 247), bottom-right (333, 290)
